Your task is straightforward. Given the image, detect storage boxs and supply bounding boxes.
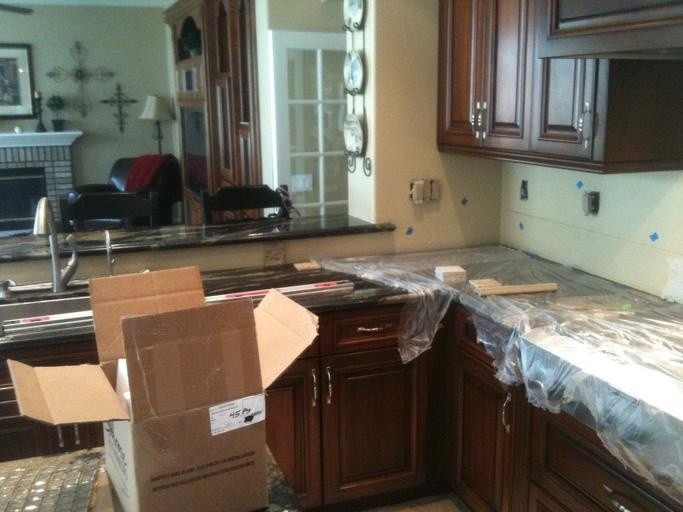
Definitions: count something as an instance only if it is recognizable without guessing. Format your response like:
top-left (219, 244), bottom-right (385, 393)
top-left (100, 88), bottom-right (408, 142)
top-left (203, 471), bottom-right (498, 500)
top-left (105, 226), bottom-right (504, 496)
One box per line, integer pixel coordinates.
top-left (6, 266), bottom-right (320, 511)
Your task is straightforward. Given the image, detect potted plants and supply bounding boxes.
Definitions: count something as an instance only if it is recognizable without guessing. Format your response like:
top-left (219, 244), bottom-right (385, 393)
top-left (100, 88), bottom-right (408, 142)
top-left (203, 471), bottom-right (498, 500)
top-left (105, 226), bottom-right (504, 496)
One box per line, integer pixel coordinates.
top-left (51, 95), bottom-right (64, 133)
top-left (46, 95), bottom-right (66, 131)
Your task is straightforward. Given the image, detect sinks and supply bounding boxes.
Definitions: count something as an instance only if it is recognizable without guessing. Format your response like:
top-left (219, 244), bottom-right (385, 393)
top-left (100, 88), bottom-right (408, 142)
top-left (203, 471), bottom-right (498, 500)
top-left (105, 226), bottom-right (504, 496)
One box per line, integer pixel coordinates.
top-left (0, 292), bottom-right (93, 336)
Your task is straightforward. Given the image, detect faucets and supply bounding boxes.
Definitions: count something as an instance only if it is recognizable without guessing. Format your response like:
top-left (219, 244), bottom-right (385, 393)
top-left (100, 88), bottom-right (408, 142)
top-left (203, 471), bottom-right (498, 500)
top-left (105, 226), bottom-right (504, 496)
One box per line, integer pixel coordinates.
top-left (33, 196), bottom-right (63, 293)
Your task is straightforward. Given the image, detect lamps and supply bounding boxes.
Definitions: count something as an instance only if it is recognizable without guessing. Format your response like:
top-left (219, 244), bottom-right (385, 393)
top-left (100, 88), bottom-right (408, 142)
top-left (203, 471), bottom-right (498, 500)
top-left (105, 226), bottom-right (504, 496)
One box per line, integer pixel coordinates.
top-left (140, 95), bottom-right (176, 155)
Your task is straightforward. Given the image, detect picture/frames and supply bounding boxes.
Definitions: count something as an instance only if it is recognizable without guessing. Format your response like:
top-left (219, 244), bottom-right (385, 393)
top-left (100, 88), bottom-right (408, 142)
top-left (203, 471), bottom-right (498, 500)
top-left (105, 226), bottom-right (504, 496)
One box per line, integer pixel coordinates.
top-left (1, 42), bottom-right (35, 119)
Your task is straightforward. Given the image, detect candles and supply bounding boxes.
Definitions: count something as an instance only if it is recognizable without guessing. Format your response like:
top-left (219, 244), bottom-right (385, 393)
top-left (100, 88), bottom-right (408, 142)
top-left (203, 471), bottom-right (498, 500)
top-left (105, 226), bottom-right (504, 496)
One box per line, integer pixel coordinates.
top-left (38, 91), bottom-right (42, 97)
top-left (34, 90), bottom-right (42, 98)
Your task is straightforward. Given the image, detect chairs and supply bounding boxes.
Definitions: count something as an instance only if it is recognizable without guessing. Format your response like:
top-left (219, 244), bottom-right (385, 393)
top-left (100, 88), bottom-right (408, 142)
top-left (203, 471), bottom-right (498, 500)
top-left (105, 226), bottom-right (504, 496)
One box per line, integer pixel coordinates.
top-left (61, 192), bottom-right (160, 231)
top-left (202, 185), bottom-right (292, 224)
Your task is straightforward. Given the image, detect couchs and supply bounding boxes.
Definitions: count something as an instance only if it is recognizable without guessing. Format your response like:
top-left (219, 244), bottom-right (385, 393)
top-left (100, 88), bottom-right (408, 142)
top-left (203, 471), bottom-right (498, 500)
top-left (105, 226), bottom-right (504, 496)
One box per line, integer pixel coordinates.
top-left (67, 158), bottom-right (182, 226)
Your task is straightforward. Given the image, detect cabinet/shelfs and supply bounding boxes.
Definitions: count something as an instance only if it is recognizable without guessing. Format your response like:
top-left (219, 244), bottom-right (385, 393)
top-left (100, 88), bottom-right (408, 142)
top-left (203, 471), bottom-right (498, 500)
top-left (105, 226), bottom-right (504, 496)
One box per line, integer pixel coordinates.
top-left (265, 302), bottom-right (431, 512)
top-left (437, 1), bottom-right (683, 175)
top-left (527, 342), bottom-right (682, 512)
top-left (0, 334), bottom-right (105, 465)
top-left (162, 0), bottom-right (263, 225)
top-left (536, 1), bottom-right (683, 61)
top-left (0, 130), bottom-right (86, 224)
top-left (441, 303), bottom-right (524, 511)
